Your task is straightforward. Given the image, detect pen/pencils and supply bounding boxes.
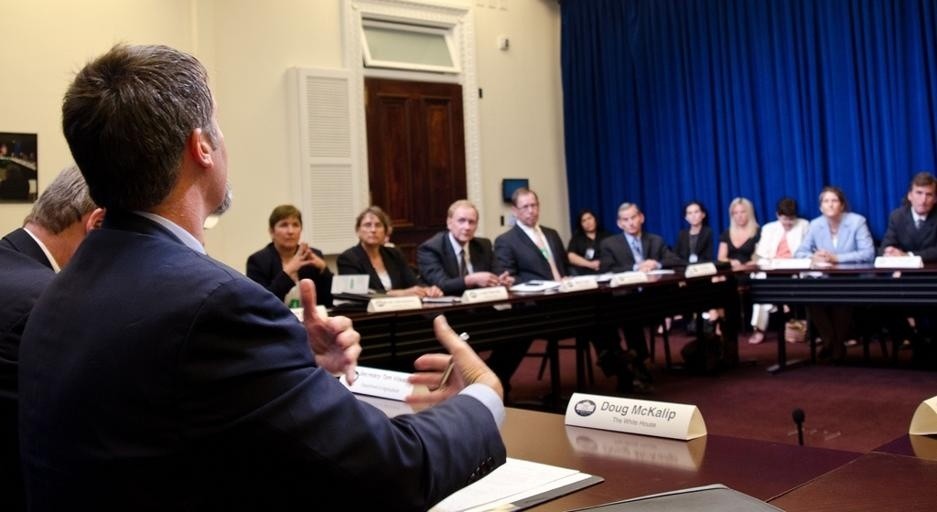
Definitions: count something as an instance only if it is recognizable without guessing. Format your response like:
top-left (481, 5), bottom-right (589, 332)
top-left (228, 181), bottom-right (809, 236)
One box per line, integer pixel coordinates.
top-left (439, 332), bottom-right (469, 392)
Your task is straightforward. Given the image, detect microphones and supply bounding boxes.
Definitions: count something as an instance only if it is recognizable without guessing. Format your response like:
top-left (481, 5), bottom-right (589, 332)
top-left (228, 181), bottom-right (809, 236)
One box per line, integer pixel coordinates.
top-left (791, 408), bottom-right (805, 445)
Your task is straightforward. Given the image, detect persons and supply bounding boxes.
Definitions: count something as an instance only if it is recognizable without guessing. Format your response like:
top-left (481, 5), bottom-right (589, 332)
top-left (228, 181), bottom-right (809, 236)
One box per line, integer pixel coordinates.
top-left (0, 44), bottom-right (507, 512)
top-left (676, 172), bottom-right (937, 351)
top-left (245, 187), bottom-right (681, 379)
top-left (0, 161), bottom-right (105, 377)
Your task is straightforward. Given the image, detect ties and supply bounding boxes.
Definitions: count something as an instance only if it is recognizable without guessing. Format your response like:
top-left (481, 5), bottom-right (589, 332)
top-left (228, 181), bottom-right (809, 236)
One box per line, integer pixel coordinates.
top-left (460, 249), bottom-right (468, 276)
top-left (633, 237), bottom-right (641, 258)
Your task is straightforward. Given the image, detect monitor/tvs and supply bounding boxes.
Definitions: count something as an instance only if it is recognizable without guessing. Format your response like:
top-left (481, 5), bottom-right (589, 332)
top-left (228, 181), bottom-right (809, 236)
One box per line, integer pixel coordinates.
top-left (0, 131), bottom-right (38, 204)
top-left (503, 179), bottom-right (528, 202)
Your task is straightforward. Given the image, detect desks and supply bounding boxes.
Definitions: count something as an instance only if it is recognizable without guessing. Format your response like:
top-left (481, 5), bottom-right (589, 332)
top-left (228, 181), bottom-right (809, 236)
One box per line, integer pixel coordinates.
top-left (325, 256), bottom-right (744, 414)
top-left (349, 390), bottom-right (937, 511)
top-left (746, 266), bottom-right (936, 376)
